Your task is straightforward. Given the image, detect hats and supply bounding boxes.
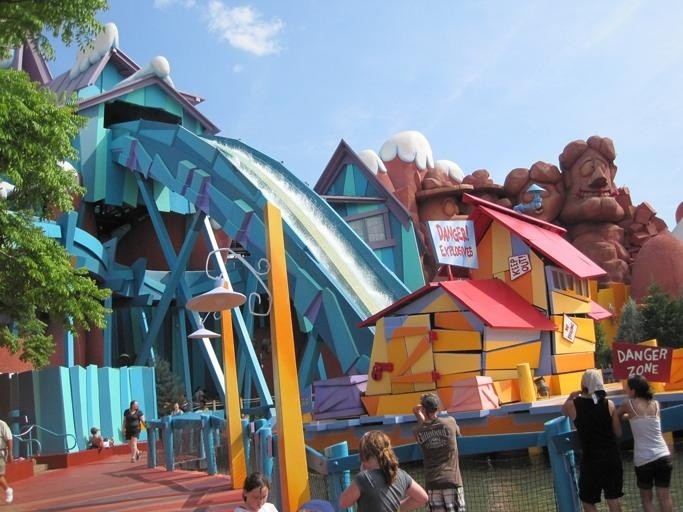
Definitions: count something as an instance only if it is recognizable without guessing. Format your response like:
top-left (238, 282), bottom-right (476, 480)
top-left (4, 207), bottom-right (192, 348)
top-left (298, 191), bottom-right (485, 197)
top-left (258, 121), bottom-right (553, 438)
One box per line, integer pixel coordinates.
top-left (421, 393), bottom-right (440, 412)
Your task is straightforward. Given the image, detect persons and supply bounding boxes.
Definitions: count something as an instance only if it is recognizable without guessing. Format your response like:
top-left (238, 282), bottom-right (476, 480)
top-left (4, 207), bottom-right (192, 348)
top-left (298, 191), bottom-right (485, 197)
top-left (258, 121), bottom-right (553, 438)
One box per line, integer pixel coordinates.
top-left (88, 427), bottom-right (114, 449)
top-left (0, 420), bottom-right (14, 503)
top-left (233, 471), bottom-right (278, 512)
top-left (200, 400), bottom-right (209, 411)
top-left (170, 402), bottom-right (184, 415)
top-left (339, 430), bottom-right (429, 512)
top-left (412, 392), bottom-right (466, 512)
top-left (122, 400), bottom-right (147, 463)
top-left (194, 386), bottom-right (208, 402)
top-left (617, 375), bottom-right (674, 512)
top-left (561, 367), bottom-right (624, 512)
top-left (203, 389), bottom-right (208, 399)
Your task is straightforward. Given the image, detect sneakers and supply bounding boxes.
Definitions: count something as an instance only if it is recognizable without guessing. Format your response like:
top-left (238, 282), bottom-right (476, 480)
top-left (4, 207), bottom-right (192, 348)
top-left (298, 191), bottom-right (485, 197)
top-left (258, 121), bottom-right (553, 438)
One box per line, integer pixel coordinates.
top-left (5, 488), bottom-right (13, 503)
top-left (131, 450), bottom-right (142, 464)
